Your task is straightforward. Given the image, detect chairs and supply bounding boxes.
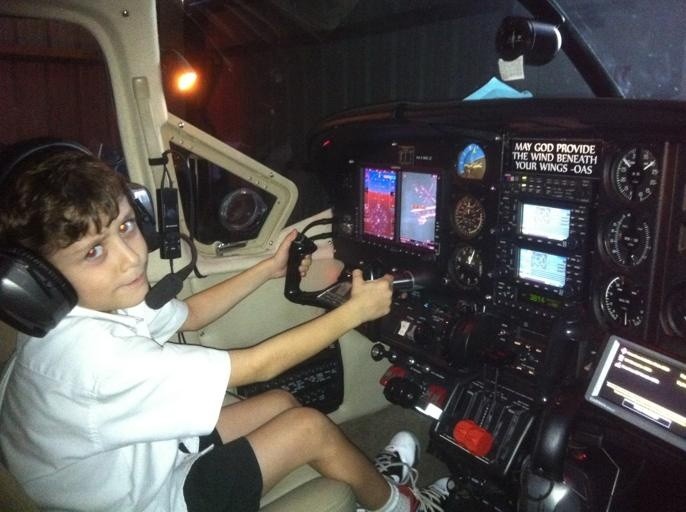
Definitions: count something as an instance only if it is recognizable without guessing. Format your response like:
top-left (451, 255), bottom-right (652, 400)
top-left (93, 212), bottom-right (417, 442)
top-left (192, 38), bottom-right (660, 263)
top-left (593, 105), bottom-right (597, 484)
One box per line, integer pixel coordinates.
top-left (0, 380), bottom-right (354, 512)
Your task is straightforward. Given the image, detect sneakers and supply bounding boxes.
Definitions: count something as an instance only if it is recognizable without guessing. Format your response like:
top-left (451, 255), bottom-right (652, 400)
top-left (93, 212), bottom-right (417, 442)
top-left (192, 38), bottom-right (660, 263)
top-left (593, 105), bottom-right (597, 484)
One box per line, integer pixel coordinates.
top-left (372, 431), bottom-right (421, 485)
top-left (399, 467), bottom-right (456, 512)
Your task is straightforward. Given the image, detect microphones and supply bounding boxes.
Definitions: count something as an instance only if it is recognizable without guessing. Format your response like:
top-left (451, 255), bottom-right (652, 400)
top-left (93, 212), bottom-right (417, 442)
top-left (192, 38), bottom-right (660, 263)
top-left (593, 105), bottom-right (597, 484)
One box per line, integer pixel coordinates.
top-left (145, 233), bottom-right (198, 310)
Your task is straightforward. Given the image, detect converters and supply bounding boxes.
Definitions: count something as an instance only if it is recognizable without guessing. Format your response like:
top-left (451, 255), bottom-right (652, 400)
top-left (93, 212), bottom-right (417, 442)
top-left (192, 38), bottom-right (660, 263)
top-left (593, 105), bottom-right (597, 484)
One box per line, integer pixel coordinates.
top-left (158, 188), bottom-right (181, 258)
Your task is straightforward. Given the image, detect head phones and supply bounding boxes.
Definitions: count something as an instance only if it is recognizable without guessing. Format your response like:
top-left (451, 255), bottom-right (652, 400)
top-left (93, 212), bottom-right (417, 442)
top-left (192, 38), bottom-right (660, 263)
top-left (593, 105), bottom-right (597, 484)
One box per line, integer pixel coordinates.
top-left (0, 137), bottom-right (155, 338)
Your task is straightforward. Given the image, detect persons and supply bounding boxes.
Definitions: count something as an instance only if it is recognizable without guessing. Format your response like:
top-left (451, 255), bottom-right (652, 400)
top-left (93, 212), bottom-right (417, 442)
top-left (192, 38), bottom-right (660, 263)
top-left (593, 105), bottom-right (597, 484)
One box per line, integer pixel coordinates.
top-left (2, 144), bottom-right (458, 511)
